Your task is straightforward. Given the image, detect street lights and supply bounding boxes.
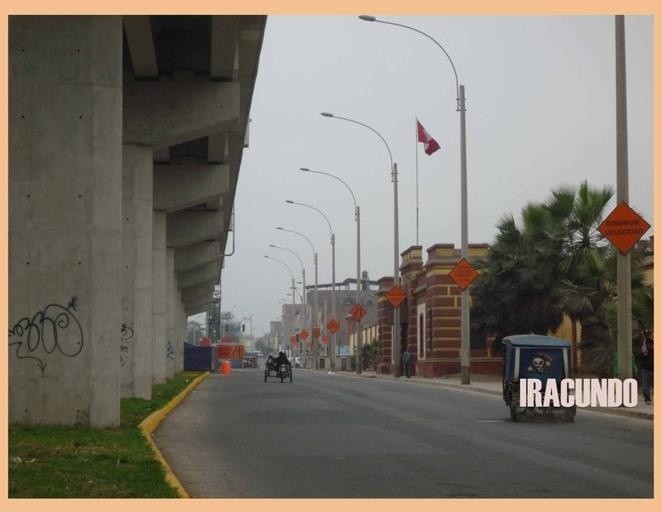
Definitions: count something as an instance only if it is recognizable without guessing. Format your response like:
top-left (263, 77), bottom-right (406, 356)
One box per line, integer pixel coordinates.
top-left (230, 305), bottom-right (255, 351)
top-left (262, 110), bottom-right (401, 367)
top-left (356, 16), bottom-right (472, 367)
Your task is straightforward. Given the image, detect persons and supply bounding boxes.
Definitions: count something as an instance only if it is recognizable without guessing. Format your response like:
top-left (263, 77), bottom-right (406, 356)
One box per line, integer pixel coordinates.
top-left (610, 328), bottom-right (654, 404)
top-left (268, 352), bottom-right (290, 365)
top-left (402, 347), bottom-right (411, 378)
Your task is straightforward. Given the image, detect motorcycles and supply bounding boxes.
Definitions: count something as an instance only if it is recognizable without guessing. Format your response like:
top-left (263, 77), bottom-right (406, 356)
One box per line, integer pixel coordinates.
top-left (501, 333), bottom-right (577, 424)
top-left (264, 360), bottom-right (293, 383)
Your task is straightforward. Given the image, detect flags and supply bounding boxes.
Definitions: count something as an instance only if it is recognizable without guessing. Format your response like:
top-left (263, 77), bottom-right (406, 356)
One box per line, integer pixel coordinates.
top-left (417, 121), bottom-right (441, 155)
